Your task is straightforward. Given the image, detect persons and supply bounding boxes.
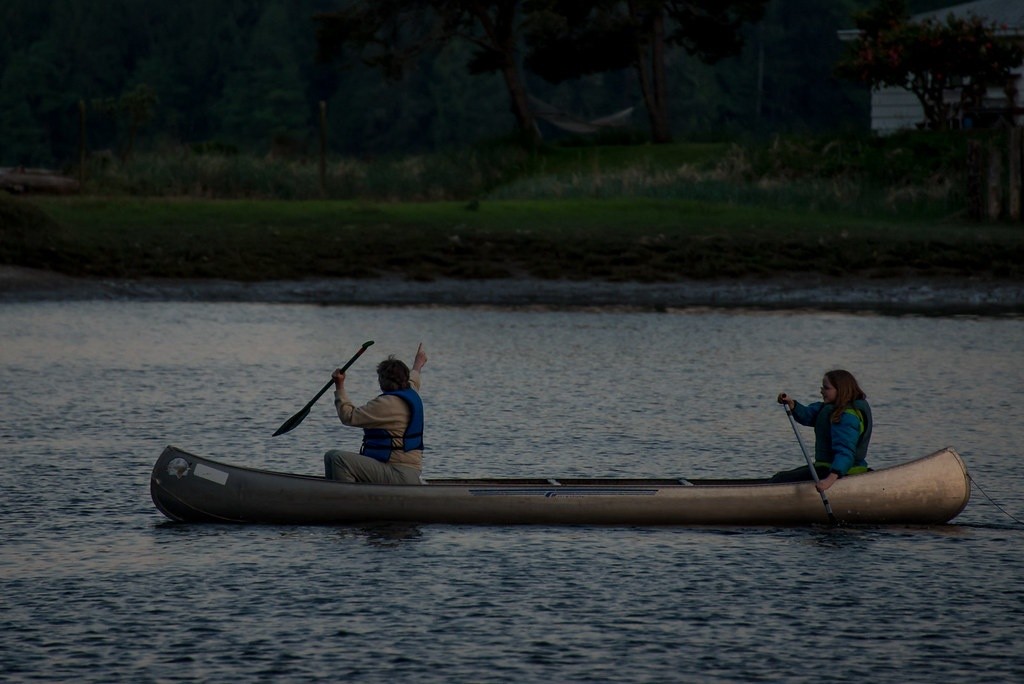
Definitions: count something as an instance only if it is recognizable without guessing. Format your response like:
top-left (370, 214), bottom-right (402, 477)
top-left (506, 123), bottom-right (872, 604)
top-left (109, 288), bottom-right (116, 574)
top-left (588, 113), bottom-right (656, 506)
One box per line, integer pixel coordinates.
top-left (770, 369), bottom-right (873, 493)
top-left (324, 342), bottom-right (428, 484)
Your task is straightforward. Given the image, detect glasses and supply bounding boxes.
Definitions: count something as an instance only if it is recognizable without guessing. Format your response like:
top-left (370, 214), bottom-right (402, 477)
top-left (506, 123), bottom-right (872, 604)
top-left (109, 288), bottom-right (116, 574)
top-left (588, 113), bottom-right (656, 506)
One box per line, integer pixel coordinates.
top-left (819, 386), bottom-right (835, 391)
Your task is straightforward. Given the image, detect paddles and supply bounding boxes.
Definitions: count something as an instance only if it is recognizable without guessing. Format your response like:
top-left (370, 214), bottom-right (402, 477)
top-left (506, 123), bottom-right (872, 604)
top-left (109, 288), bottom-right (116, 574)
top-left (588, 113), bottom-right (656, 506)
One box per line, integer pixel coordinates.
top-left (781, 393), bottom-right (841, 526)
top-left (271, 339), bottom-right (376, 438)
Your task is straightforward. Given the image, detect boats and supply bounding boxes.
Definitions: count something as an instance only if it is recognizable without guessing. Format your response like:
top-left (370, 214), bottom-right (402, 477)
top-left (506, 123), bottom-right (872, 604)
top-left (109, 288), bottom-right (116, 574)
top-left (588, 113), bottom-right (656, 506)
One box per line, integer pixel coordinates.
top-left (150, 444), bottom-right (969, 526)
